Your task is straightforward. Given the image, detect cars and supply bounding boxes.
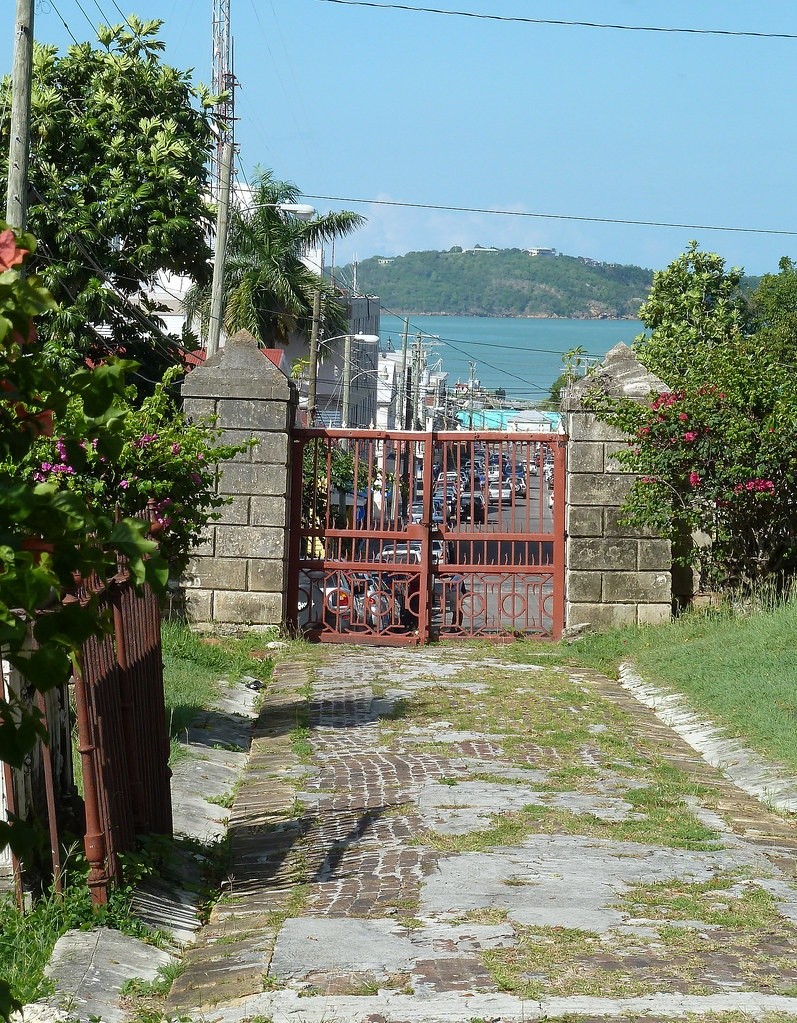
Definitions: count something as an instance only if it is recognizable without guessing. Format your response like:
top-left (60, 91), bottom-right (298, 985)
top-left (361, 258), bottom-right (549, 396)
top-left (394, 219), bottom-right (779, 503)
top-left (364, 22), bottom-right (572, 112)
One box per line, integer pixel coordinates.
top-left (468, 445), bottom-right (555, 520)
top-left (300, 471), bottom-right (482, 623)
top-left (523, 440), bottom-right (553, 509)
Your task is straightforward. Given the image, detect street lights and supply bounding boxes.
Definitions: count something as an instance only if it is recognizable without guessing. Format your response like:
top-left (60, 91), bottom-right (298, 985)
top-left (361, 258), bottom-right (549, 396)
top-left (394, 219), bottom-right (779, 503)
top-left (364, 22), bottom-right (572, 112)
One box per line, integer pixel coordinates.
top-left (340, 333), bottom-right (383, 503)
top-left (309, 328), bottom-right (379, 428)
top-left (207, 200), bottom-right (315, 364)
